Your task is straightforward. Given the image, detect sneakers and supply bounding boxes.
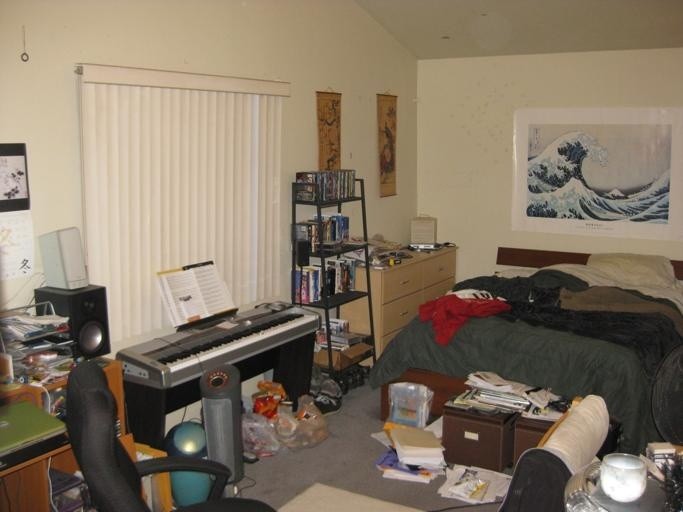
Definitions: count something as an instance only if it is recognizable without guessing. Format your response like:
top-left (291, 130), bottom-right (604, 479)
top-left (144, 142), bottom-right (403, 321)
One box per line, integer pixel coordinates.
top-left (314, 396), bottom-right (341, 417)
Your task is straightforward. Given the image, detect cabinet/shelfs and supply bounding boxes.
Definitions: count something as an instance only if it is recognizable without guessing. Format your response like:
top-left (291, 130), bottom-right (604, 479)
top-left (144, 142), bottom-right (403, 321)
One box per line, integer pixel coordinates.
top-left (339, 244), bottom-right (459, 360)
top-left (290, 178), bottom-right (377, 394)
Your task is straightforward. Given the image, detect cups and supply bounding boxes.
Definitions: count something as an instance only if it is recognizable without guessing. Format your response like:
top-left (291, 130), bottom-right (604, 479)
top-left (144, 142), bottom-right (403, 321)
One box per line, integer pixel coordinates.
top-left (600, 452), bottom-right (648, 503)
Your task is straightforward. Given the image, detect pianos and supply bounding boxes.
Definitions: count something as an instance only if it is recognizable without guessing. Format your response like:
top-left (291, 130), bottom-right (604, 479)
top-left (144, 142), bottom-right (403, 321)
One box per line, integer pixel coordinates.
top-left (116, 302), bottom-right (321, 389)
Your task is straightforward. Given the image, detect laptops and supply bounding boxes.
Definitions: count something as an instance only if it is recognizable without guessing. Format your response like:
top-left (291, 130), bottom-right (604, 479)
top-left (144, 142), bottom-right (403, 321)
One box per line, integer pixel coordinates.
top-left (0, 399), bottom-right (67, 458)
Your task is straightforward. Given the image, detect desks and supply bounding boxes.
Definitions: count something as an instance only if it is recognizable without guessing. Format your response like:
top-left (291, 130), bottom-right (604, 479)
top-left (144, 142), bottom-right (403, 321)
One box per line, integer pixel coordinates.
top-left (0, 356), bottom-right (127, 512)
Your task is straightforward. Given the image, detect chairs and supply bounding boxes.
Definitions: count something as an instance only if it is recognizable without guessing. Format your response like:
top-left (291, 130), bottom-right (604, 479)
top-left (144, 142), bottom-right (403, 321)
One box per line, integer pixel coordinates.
top-left (65, 361), bottom-right (278, 512)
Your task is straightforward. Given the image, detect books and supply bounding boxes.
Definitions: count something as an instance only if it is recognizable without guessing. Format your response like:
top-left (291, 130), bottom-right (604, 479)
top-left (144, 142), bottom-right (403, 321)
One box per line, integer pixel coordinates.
top-left (294, 257), bottom-right (355, 303)
top-left (369, 370), bottom-right (563, 484)
top-left (158, 264), bottom-right (236, 326)
top-left (296, 213), bottom-right (371, 253)
top-left (114, 418), bottom-right (121, 438)
top-left (295, 169), bottom-right (356, 202)
top-left (318, 319), bottom-right (367, 351)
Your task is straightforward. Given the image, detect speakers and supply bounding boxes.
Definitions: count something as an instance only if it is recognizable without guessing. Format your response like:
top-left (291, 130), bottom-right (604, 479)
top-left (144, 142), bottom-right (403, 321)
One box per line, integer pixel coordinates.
top-left (200, 364), bottom-right (244, 484)
top-left (40, 226), bottom-right (88, 290)
top-left (34, 284), bottom-right (111, 359)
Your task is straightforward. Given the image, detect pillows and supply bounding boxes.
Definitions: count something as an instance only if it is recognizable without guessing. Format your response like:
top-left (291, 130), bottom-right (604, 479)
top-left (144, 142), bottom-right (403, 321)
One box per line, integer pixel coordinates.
top-left (585, 252), bottom-right (679, 292)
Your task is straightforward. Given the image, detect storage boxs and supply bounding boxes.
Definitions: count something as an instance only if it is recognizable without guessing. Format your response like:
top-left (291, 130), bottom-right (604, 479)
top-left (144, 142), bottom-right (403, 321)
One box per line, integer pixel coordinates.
top-left (441, 397), bottom-right (622, 476)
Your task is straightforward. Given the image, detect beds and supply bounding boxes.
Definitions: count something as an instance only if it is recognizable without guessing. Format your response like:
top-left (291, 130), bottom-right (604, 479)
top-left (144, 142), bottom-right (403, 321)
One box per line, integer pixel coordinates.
top-left (413, 247), bottom-right (682, 432)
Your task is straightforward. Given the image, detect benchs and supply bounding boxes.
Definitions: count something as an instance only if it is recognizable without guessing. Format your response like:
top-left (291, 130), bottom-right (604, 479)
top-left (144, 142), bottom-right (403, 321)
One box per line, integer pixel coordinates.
top-left (381, 367), bottom-right (472, 427)
